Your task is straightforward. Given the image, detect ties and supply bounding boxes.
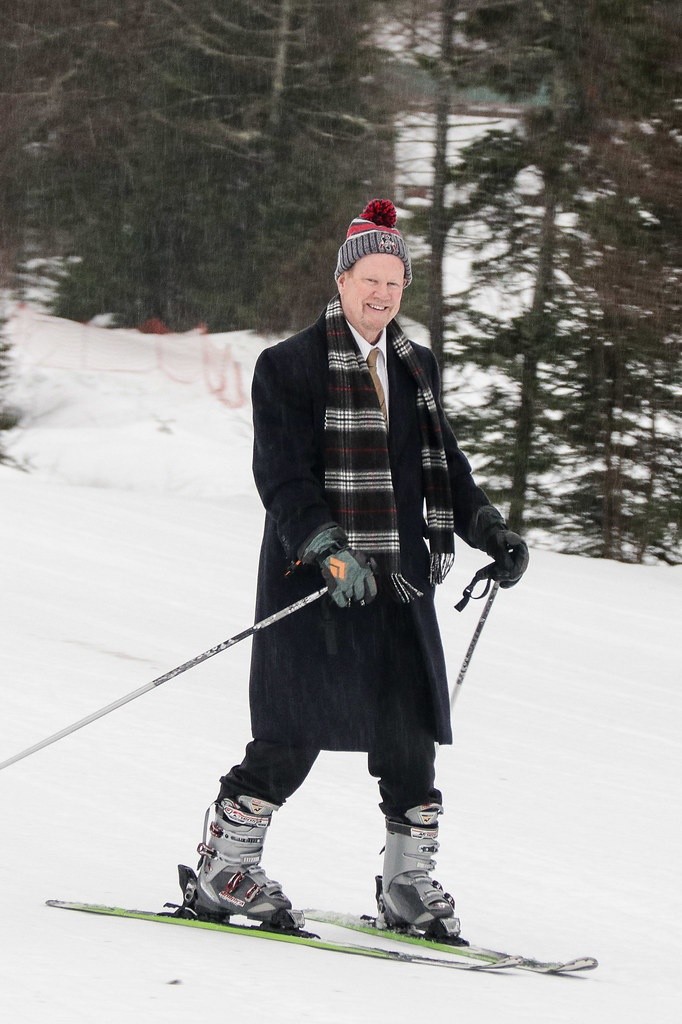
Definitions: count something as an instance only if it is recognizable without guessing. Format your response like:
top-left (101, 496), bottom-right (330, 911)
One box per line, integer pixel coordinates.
top-left (366, 349), bottom-right (387, 420)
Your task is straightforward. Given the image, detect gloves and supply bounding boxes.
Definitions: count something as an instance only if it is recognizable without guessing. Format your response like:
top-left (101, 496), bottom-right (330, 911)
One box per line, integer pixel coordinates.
top-left (473, 506), bottom-right (529, 587)
top-left (297, 522), bottom-right (377, 607)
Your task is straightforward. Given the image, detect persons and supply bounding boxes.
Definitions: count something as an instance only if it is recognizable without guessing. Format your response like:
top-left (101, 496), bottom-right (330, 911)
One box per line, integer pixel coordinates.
top-left (196, 200), bottom-right (529, 940)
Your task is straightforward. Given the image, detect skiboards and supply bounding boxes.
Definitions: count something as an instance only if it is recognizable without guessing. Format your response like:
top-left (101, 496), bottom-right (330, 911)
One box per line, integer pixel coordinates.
top-left (44, 898), bottom-right (598, 978)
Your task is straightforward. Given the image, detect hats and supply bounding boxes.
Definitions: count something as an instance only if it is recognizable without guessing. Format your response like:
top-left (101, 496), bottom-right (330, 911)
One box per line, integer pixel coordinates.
top-left (334, 199), bottom-right (412, 289)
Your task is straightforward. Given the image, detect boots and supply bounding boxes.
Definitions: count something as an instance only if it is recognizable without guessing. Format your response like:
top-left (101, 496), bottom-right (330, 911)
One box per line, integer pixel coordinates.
top-left (376, 802), bottom-right (455, 930)
top-left (178, 791), bottom-right (292, 919)
